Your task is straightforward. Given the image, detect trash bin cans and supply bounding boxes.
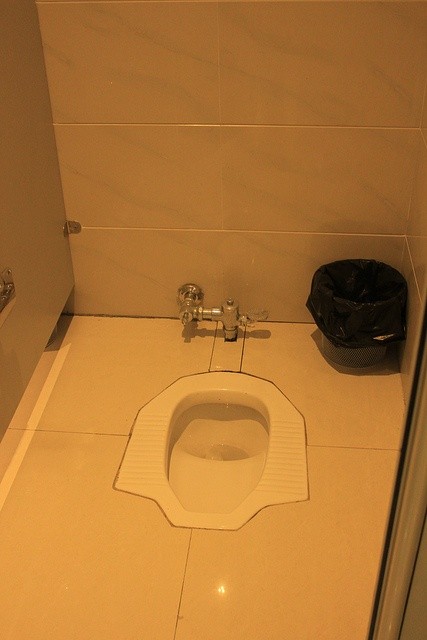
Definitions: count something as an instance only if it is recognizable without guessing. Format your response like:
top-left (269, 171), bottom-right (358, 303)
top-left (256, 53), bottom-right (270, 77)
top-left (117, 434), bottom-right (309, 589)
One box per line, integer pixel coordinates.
top-left (315, 259), bottom-right (406, 368)
